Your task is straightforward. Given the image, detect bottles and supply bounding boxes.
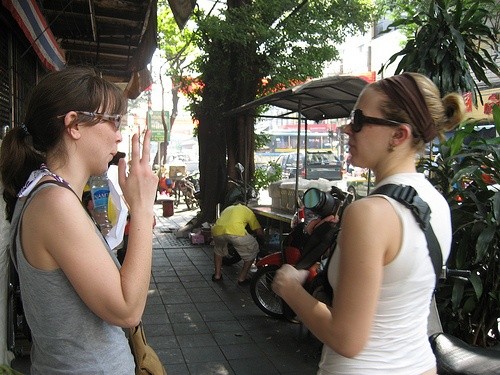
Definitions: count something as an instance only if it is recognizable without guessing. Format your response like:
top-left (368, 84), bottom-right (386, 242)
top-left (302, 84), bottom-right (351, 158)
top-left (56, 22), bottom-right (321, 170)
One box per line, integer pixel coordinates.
top-left (90, 171), bottom-right (112, 238)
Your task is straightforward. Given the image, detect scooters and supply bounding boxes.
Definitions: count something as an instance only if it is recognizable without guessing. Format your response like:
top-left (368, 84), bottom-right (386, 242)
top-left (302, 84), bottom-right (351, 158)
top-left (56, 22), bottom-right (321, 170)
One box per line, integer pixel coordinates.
top-left (250, 184), bottom-right (500, 375)
top-left (155, 172), bottom-right (200, 210)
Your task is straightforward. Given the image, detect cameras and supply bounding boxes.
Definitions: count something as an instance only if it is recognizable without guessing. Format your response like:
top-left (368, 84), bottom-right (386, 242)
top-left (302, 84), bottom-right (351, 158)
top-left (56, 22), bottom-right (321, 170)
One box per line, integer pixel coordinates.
top-left (303, 186), bottom-right (366, 239)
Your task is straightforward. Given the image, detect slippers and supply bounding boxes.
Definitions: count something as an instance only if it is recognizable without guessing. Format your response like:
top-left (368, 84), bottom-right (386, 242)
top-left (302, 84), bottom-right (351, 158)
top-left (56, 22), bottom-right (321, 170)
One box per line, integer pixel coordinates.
top-left (212, 273), bottom-right (222, 282)
top-left (237, 277), bottom-right (253, 286)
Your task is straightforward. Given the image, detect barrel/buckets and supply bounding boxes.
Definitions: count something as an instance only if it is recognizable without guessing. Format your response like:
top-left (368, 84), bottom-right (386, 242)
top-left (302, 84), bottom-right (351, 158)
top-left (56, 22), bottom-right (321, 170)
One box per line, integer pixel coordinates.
top-left (162, 199), bottom-right (175, 217)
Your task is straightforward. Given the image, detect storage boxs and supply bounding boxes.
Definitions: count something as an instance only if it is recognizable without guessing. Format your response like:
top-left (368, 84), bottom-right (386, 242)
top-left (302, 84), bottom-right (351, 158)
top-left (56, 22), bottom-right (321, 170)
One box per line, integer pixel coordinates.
top-left (271, 181), bottom-right (305, 212)
top-left (191, 233), bottom-right (205, 244)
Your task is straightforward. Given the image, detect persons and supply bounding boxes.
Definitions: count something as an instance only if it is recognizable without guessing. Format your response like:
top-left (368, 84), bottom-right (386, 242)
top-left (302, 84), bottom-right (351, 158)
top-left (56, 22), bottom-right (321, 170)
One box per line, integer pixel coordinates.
top-left (273, 72), bottom-right (466, 375)
top-left (0, 64), bottom-right (167, 375)
top-left (211, 193), bottom-right (265, 284)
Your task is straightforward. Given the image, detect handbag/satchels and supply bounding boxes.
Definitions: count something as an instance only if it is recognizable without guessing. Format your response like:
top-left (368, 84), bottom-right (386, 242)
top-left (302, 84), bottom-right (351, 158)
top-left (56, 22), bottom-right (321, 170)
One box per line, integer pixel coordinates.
top-left (127, 319), bottom-right (166, 375)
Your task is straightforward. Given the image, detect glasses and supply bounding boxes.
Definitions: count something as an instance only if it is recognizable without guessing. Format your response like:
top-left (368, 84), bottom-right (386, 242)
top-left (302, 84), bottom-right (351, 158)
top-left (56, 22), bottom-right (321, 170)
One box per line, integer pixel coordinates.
top-left (57, 111), bottom-right (122, 130)
top-left (350, 109), bottom-right (419, 139)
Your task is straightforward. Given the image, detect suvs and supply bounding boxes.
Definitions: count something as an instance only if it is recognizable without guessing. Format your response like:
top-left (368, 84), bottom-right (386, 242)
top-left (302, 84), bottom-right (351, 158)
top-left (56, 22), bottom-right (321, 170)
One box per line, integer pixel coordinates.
top-left (266, 150), bottom-right (343, 181)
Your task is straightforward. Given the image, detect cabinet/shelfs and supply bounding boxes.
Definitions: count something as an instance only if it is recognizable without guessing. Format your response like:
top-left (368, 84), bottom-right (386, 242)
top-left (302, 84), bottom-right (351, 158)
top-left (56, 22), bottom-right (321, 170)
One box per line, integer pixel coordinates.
top-left (252, 207), bottom-right (293, 255)
